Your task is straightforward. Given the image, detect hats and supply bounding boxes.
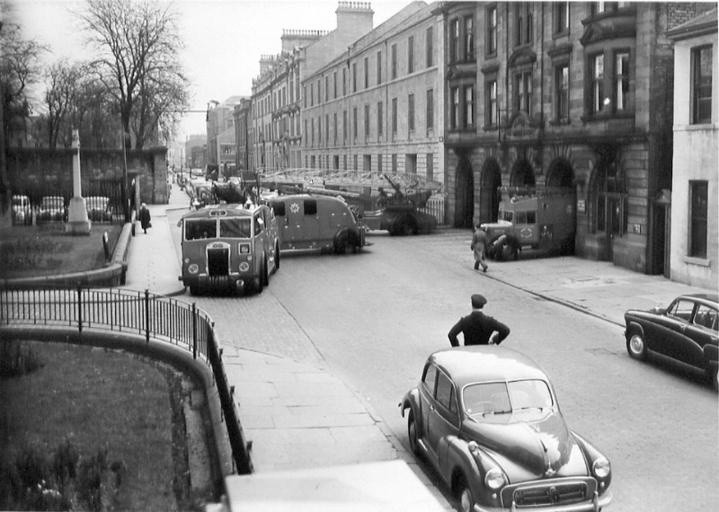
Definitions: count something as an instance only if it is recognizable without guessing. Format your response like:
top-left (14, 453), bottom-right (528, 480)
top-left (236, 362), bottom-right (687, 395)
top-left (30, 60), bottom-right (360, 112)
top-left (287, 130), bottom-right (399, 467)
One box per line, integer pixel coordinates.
top-left (471, 294), bottom-right (486, 305)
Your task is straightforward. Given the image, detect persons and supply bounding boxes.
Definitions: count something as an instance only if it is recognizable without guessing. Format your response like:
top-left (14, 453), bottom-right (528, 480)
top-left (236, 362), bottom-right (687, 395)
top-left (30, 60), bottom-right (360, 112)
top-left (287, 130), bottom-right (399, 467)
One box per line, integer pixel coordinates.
top-left (448, 295), bottom-right (510, 347)
top-left (139, 203), bottom-right (150, 234)
top-left (470, 224), bottom-right (488, 272)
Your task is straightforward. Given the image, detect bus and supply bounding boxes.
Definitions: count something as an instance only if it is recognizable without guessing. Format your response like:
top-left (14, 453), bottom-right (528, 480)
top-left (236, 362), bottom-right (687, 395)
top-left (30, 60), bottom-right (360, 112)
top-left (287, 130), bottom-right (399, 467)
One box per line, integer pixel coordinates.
top-left (178, 203), bottom-right (280, 297)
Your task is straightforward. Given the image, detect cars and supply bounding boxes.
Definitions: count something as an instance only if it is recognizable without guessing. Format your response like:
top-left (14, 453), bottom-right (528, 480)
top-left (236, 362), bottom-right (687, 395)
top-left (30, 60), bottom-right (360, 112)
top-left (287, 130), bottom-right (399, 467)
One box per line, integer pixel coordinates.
top-left (176, 162), bottom-right (259, 205)
top-left (12, 194), bottom-right (116, 225)
top-left (623, 293), bottom-right (719, 386)
top-left (397, 342), bottom-right (614, 512)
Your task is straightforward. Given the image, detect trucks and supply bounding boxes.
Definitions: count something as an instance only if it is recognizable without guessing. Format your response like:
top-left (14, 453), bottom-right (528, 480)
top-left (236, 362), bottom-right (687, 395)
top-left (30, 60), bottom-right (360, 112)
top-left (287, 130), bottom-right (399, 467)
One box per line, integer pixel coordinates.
top-left (266, 193), bottom-right (370, 258)
top-left (342, 175), bottom-right (440, 235)
top-left (474, 184), bottom-right (574, 261)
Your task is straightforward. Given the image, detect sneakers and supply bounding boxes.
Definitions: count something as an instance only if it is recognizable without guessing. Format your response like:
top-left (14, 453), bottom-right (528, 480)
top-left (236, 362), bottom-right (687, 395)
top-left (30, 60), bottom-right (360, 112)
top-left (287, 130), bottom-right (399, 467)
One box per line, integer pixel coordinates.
top-left (483, 266), bottom-right (488, 272)
